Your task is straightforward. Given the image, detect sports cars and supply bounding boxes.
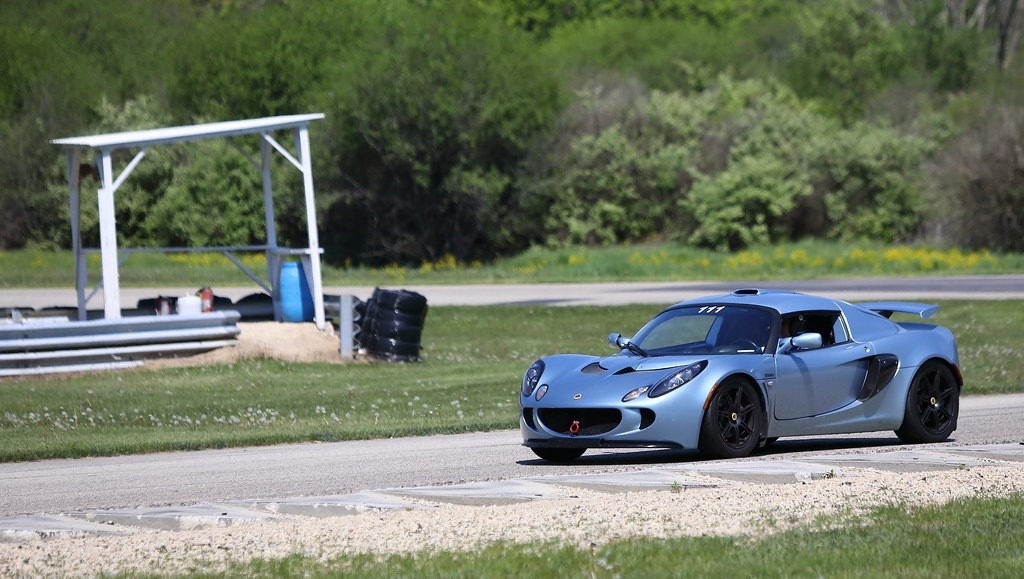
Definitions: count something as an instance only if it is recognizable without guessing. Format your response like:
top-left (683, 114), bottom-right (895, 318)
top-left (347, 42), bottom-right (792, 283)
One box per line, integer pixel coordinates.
top-left (517, 287), bottom-right (964, 464)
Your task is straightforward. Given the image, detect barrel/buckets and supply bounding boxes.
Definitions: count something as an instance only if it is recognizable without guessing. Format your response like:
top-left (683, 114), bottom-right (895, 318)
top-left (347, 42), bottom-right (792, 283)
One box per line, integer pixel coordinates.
top-left (280, 262), bottom-right (314, 322)
top-left (176, 296), bottom-right (202, 314)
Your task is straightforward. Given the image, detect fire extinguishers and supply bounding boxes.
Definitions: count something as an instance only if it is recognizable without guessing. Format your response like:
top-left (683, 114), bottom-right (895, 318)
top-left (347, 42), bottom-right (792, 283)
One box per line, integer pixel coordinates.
top-left (196, 285), bottom-right (213, 313)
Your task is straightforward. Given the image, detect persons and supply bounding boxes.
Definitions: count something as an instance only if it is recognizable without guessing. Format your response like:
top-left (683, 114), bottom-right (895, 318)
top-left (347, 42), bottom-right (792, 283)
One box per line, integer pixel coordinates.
top-left (756, 315), bottom-right (806, 354)
